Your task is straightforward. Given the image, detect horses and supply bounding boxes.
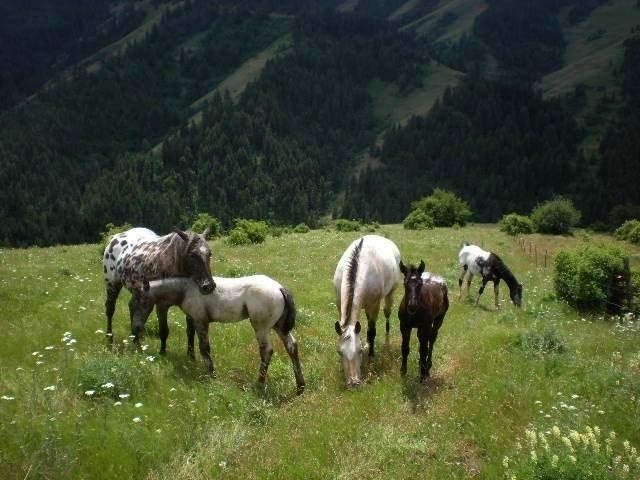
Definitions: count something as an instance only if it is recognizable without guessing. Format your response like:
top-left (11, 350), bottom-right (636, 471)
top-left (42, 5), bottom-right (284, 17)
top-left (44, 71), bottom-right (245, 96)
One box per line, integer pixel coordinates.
top-left (128, 273), bottom-right (307, 396)
top-left (331, 234), bottom-right (402, 390)
top-left (397, 259), bottom-right (450, 384)
top-left (102, 223), bottom-right (217, 360)
top-left (457, 240), bottom-right (523, 307)
